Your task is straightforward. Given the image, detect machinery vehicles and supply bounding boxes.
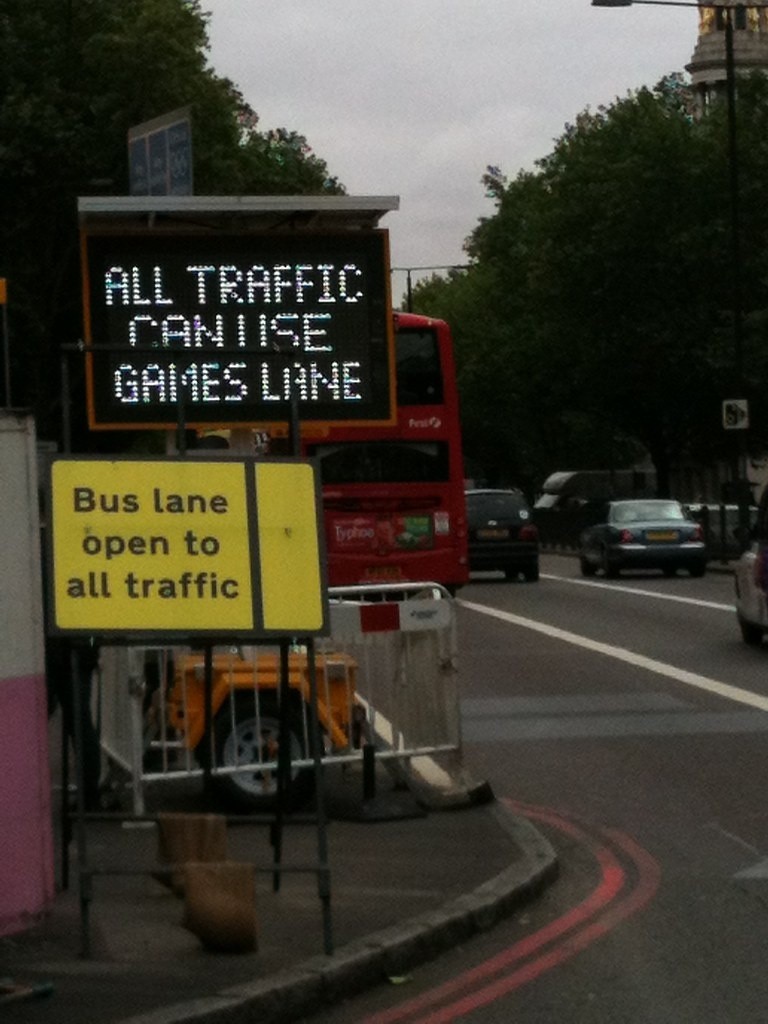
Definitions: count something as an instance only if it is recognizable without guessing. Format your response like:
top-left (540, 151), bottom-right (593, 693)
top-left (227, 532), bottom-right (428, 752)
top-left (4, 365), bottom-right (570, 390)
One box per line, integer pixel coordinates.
top-left (44, 621), bottom-right (364, 822)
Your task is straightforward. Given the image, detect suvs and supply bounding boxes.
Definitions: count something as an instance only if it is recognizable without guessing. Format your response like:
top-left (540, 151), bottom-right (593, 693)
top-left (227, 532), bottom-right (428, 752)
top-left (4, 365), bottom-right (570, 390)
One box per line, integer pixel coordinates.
top-left (465, 486), bottom-right (541, 585)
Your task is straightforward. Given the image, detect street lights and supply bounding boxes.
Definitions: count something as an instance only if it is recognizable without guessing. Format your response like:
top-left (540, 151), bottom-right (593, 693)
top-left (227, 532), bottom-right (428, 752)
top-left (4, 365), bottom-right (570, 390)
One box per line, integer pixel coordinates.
top-left (590, 0), bottom-right (743, 493)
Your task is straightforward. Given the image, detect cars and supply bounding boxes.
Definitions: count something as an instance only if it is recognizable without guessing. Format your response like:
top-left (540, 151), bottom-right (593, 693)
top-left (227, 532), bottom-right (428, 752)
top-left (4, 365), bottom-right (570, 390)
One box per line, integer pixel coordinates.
top-left (578, 500), bottom-right (709, 579)
top-left (731, 527), bottom-right (768, 644)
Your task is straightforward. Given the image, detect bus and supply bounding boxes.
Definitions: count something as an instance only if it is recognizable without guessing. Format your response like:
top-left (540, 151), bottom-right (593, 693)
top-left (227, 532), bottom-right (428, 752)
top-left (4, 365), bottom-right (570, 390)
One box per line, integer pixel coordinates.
top-left (250, 314), bottom-right (469, 609)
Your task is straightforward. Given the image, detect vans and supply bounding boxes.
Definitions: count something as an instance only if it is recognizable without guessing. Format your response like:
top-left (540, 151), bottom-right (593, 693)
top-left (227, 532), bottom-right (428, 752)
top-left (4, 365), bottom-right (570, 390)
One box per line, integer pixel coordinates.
top-left (686, 502), bottom-right (765, 553)
